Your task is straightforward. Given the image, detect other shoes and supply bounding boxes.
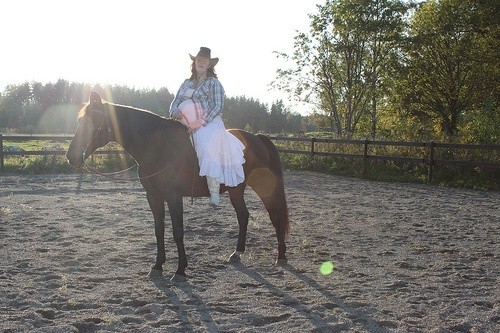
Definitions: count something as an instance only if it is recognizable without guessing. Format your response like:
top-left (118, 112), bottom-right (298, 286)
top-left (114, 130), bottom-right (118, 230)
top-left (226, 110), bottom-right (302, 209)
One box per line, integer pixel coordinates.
top-left (209, 192), bottom-right (220, 207)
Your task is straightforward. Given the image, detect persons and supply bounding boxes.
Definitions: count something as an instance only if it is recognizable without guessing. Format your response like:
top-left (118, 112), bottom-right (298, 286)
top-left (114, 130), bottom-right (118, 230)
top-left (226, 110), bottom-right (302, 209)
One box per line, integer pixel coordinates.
top-left (169, 47), bottom-right (246, 206)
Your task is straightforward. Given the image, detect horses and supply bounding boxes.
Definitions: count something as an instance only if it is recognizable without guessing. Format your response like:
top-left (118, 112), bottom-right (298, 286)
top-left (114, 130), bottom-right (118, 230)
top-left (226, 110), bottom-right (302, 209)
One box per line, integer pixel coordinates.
top-left (67, 91), bottom-right (290, 283)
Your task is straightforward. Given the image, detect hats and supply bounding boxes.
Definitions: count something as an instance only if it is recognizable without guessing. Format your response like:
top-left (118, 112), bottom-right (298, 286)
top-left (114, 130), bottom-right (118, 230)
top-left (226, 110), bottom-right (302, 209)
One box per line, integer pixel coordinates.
top-left (188, 47), bottom-right (219, 67)
